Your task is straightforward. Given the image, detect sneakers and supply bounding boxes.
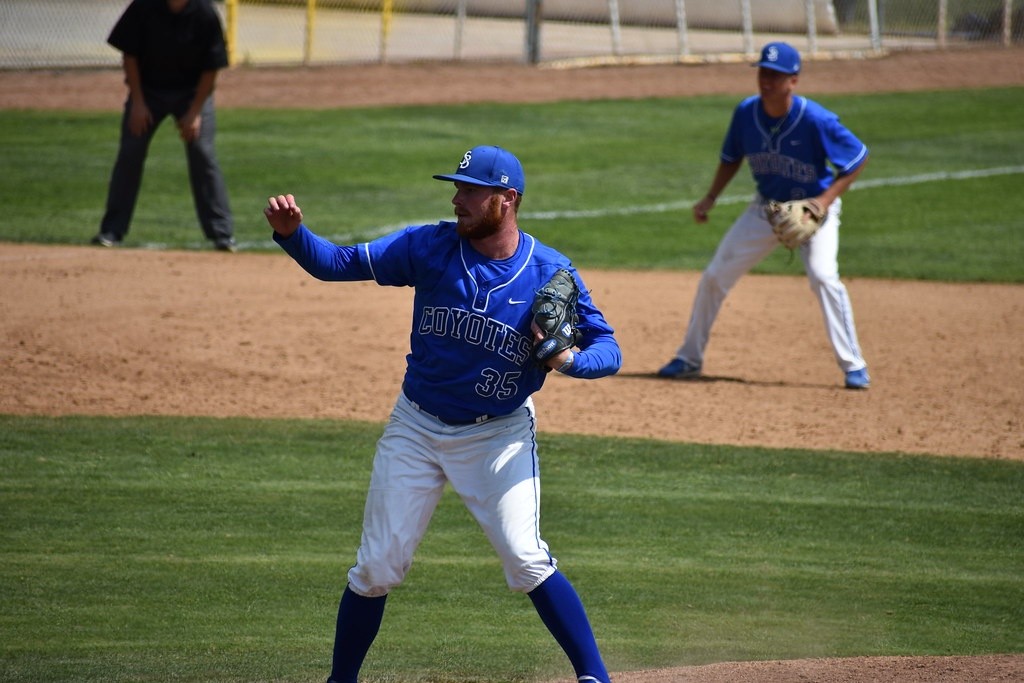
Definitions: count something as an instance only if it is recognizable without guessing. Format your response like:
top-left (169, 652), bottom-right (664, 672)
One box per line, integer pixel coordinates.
top-left (659, 359), bottom-right (701, 378)
top-left (845, 367), bottom-right (871, 389)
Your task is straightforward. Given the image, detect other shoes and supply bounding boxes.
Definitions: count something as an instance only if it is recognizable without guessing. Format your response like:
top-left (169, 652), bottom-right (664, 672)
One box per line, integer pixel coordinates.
top-left (216, 237), bottom-right (237, 252)
top-left (93, 231), bottom-right (123, 246)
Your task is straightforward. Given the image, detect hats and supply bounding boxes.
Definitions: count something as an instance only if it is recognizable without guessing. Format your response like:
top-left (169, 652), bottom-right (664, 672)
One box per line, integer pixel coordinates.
top-left (753, 42), bottom-right (801, 75)
top-left (432, 144), bottom-right (525, 196)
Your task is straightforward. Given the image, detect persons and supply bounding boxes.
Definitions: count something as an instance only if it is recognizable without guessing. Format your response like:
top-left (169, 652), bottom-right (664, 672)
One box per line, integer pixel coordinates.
top-left (92, 0), bottom-right (238, 252)
top-left (659, 42), bottom-right (870, 389)
top-left (264, 146), bottom-right (621, 683)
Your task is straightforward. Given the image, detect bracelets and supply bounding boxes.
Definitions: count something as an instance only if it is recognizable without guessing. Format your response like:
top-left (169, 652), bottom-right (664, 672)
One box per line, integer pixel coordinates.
top-left (557, 352), bottom-right (573, 372)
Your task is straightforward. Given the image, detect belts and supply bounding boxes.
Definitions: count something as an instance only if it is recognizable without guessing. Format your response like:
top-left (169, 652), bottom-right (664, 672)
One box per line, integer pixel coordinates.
top-left (402, 385), bottom-right (494, 426)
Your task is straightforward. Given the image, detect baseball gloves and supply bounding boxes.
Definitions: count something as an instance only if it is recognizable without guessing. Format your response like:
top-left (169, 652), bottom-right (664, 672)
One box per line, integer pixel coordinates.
top-left (533, 270), bottom-right (580, 368)
top-left (764, 198), bottom-right (827, 248)
